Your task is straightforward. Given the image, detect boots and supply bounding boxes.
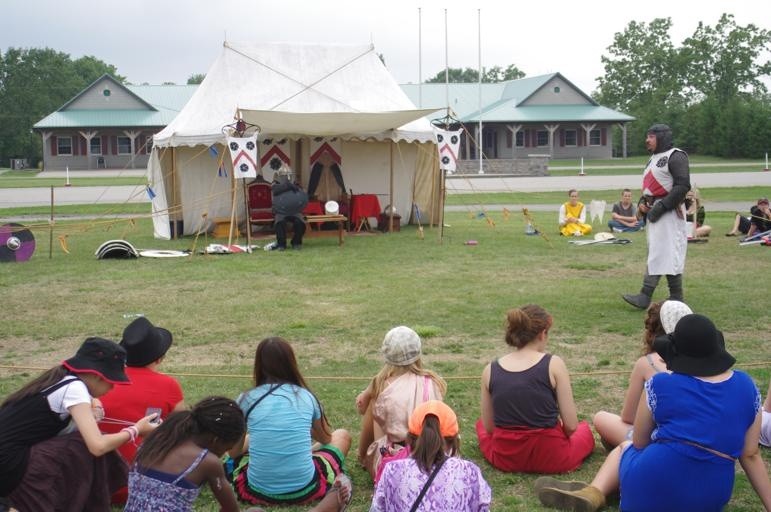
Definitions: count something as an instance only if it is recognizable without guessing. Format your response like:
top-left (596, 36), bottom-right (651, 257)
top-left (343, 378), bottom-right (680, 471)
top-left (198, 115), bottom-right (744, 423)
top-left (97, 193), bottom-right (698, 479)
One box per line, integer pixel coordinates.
top-left (533, 477), bottom-right (588, 494)
top-left (538, 484), bottom-right (607, 512)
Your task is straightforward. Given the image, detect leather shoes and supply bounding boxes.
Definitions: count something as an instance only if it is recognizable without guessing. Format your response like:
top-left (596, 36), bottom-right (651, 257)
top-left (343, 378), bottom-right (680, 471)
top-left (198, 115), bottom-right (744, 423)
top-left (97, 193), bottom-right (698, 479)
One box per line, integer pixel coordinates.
top-left (623, 294), bottom-right (651, 309)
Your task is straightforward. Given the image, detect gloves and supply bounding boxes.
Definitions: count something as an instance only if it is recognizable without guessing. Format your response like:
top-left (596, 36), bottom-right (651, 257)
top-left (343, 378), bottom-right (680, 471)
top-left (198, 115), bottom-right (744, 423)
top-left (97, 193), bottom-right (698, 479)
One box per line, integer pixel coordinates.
top-left (647, 201), bottom-right (666, 223)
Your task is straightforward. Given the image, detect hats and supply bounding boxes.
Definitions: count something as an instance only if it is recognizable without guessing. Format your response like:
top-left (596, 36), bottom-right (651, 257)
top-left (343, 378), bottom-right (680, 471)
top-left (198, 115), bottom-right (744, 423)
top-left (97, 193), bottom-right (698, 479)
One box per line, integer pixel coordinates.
top-left (659, 299), bottom-right (694, 334)
top-left (381, 325), bottom-right (422, 366)
top-left (408, 399), bottom-right (459, 437)
top-left (655, 313), bottom-right (736, 376)
top-left (757, 197), bottom-right (768, 204)
top-left (63, 337), bottom-right (133, 386)
top-left (119, 316), bottom-right (173, 367)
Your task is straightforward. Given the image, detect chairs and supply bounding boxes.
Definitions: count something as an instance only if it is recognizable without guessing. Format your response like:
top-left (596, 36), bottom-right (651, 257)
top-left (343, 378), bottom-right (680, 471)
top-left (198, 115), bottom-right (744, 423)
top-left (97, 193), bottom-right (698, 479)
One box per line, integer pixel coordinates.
top-left (245, 183), bottom-right (275, 249)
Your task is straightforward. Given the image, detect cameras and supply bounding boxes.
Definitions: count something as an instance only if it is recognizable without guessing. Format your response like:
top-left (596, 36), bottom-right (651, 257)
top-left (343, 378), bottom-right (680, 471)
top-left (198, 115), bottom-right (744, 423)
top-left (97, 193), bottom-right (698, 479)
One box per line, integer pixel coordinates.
top-left (144, 407), bottom-right (163, 424)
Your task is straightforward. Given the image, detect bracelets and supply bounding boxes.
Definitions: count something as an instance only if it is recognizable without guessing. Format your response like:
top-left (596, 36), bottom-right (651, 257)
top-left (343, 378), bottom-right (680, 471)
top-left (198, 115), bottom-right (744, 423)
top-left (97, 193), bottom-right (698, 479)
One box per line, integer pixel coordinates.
top-left (120, 428), bottom-right (134, 443)
top-left (128, 426), bottom-right (139, 438)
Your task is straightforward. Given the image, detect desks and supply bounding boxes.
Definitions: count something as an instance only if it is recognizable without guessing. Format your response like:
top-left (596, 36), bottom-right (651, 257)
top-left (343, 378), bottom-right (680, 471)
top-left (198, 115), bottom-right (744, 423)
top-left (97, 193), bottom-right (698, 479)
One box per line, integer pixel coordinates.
top-left (352, 195), bottom-right (381, 233)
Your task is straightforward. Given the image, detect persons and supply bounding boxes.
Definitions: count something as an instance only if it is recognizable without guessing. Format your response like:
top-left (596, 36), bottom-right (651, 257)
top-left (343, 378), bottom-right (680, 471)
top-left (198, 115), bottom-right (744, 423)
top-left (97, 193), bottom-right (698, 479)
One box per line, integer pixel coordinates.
top-left (621, 123), bottom-right (691, 310)
top-left (96, 317), bottom-right (186, 504)
top-left (759, 379), bottom-right (771, 446)
top-left (369, 401), bottom-right (492, 512)
top-left (124, 396), bottom-right (353, 512)
top-left (535, 314), bottom-right (771, 512)
top-left (558, 186), bottom-right (771, 243)
top-left (476, 303), bottom-right (595, 473)
top-left (355, 326), bottom-right (448, 481)
top-left (592, 300), bottom-right (694, 451)
top-left (223, 338), bottom-right (352, 508)
top-left (271, 165), bottom-right (307, 252)
top-left (0, 336), bottom-right (163, 512)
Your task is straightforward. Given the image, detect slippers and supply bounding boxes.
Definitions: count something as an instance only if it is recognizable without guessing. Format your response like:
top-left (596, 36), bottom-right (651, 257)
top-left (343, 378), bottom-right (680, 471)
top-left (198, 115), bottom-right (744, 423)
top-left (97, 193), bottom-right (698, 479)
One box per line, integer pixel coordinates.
top-left (325, 473), bottom-right (353, 512)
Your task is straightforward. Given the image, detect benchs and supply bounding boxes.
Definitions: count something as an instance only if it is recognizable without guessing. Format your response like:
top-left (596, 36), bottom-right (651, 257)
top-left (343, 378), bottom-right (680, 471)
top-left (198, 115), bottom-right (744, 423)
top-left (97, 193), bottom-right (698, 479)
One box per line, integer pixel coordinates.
top-left (304, 215), bottom-right (347, 247)
